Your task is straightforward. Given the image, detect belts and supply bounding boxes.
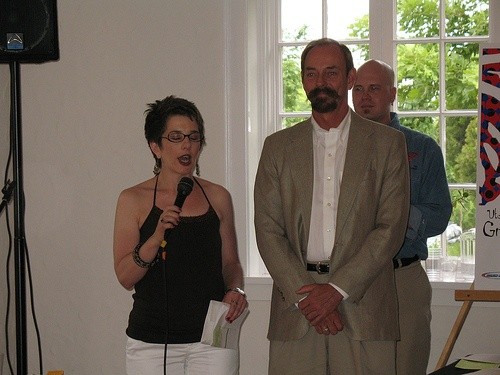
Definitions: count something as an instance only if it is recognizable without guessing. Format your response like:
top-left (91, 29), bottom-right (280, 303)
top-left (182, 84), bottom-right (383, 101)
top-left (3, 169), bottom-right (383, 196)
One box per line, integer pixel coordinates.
top-left (392, 255), bottom-right (420, 268)
top-left (307, 262), bottom-right (332, 274)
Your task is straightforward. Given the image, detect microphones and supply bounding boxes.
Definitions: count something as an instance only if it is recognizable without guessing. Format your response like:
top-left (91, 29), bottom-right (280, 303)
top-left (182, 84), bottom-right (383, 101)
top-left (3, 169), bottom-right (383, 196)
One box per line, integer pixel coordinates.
top-left (166, 176), bottom-right (194, 235)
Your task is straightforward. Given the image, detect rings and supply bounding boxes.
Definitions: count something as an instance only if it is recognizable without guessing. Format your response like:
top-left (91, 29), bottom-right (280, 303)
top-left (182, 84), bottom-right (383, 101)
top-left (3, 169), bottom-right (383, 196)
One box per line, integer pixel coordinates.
top-left (322, 328), bottom-right (329, 332)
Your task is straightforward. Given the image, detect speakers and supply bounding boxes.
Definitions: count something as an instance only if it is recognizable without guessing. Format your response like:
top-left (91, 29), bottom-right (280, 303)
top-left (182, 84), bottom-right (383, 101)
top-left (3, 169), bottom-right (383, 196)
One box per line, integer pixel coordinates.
top-left (0, 0), bottom-right (60, 64)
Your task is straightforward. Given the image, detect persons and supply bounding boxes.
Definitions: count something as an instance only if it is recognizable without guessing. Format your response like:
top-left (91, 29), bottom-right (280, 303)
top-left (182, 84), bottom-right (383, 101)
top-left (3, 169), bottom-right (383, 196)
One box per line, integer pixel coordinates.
top-left (255, 38), bottom-right (452, 375)
top-left (113, 95), bottom-right (248, 375)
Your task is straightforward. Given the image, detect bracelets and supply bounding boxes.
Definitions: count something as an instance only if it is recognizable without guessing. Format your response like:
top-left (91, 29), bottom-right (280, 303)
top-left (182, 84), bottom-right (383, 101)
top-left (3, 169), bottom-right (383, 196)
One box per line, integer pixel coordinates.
top-left (132, 240), bottom-right (161, 268)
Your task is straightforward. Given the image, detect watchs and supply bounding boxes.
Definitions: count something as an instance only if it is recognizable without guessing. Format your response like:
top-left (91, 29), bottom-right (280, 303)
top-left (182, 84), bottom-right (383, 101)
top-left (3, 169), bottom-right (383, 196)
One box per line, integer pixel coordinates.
top-left (224, 286), bottom-right (247, 296)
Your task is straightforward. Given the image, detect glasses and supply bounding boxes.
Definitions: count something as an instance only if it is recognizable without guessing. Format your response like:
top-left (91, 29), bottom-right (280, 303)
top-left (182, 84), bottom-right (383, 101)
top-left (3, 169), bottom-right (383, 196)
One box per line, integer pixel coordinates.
top-left (159, 131), bottom-right (205, 143)
